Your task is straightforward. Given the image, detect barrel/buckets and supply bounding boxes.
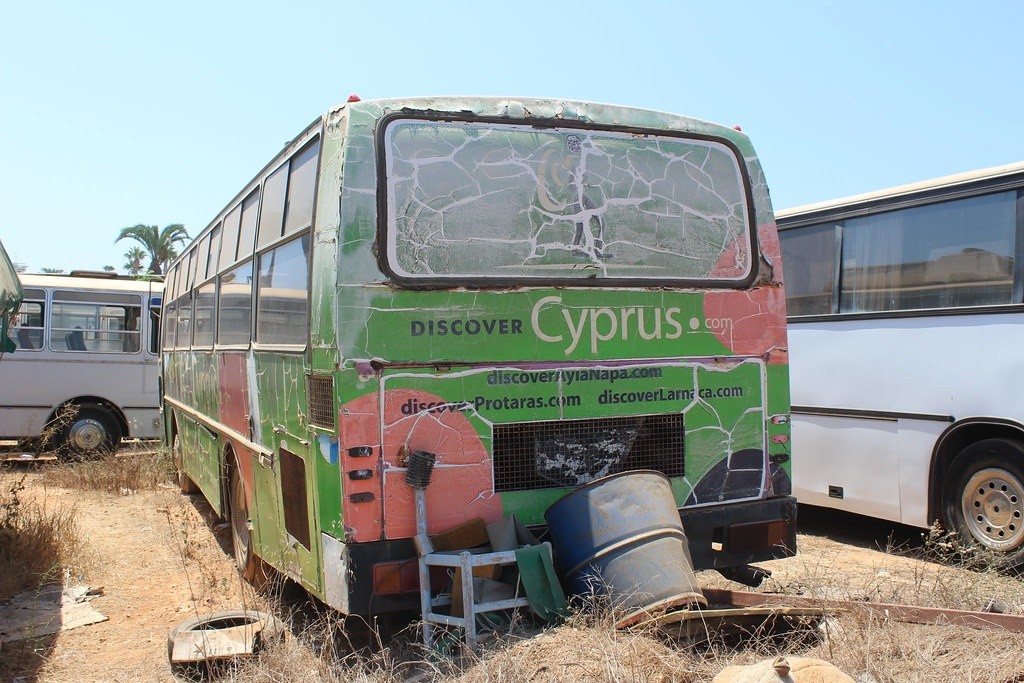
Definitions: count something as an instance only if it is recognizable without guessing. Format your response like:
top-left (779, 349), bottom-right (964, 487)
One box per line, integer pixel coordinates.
top-left (544, 469), bottom-right (708, 633)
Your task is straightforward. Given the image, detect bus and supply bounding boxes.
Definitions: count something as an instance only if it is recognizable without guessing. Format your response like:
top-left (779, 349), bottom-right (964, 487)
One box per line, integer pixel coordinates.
top-left (0, 270), bottom-right (165, 463)
top-left (774, 162), bottom-right (1024, 574)
top-left (156, 93), bottom-right (798, 619)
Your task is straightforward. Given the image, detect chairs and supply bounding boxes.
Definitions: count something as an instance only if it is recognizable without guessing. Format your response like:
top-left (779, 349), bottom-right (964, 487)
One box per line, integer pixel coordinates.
top-left (65, 326), bottom-right (87, 351)
top-left (18, 323), bottom-right (35, 349)
top-left (127, 317), bottom-right (140, 346)
